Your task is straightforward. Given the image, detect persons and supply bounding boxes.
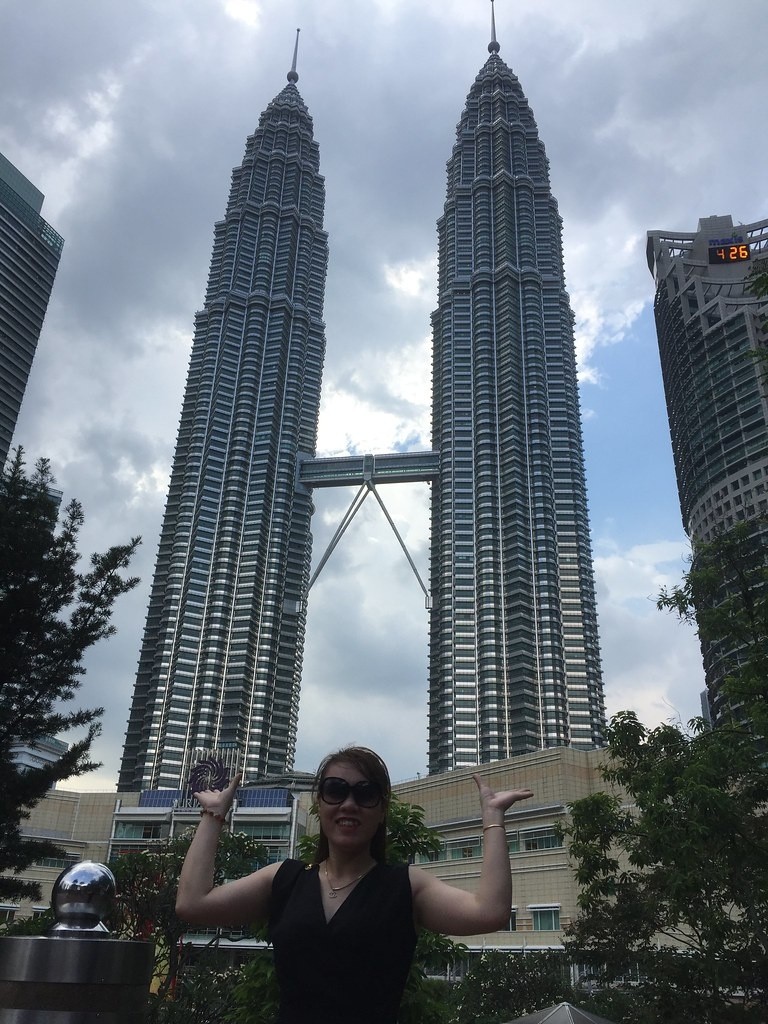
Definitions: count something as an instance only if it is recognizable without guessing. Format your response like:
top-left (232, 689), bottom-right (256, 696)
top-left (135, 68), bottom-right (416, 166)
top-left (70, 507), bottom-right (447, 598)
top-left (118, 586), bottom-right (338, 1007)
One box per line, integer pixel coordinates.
top-left (175, 747), bottom-right (534, 1024)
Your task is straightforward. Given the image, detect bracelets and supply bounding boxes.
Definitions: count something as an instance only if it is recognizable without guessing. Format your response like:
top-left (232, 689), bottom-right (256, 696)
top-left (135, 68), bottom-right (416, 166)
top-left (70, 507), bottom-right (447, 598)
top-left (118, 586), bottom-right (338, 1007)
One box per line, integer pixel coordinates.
top-left (201, 808), bottom-right (227, 825)
top-left (483, 824), bottom-right (506, 833)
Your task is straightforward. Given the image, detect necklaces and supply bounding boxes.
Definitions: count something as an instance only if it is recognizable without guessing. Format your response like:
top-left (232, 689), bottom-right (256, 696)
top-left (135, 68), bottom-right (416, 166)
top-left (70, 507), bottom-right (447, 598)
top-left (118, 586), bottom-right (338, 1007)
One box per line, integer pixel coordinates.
top-left (325, 859), bottom-right (378, 898)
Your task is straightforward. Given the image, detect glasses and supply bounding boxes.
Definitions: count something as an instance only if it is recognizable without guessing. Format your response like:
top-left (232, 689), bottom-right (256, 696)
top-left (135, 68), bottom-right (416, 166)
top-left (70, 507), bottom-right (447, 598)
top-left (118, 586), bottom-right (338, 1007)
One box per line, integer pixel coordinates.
top-left (315, 777), bottom-right (386, 809)
